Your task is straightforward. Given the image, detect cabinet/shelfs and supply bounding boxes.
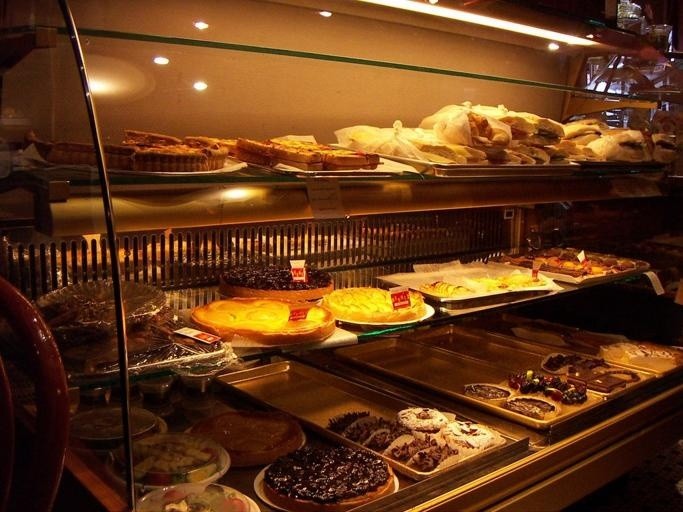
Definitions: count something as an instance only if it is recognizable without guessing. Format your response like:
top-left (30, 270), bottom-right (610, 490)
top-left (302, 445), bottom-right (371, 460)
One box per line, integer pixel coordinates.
top-left (0, 0), bottom-right (683, 512)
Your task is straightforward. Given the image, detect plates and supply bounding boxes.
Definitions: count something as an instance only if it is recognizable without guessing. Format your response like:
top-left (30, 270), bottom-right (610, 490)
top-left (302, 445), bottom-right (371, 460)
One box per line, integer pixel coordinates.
top-left (252, 463), bottom-right (400, 512)
top-left (105, 444), bottom-right (230, 492)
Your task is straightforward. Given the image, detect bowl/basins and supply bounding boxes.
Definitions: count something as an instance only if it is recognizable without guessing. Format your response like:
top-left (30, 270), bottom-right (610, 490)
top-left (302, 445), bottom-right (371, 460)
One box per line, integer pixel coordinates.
top-left (137, 372), bottom-right (215, 429)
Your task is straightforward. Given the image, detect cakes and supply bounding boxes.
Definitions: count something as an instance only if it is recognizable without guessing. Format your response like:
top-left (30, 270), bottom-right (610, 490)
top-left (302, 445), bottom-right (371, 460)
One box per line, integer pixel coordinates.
top-left (439, 421), bottom-right (494, 449)
top-left (219, 264), bottom-right (335, 301)
top-left (68, 406), bottom-right (160, 450)
top-left (326, 410), bottom-right (395, 459)
top-left (540, 351), bottom-right (642, 393)
top-left (262, 447), bottom-right (395, 512)
top-left (190, 296), bottom-right (337, 345)
top-left (322, 286), bottom-right (426, 323)
top-left (31, 280), bottom-right (174, 368)
top-left (464, 383), bottom-right (515, 408)
top-left (112, 434), bottom-right (224, 486)
top-left (508, 369), bottom-right (544, 394)
top-left (395, 435), bottom-right (459, 472)
top-left (544, 376), bottom-right (587, 405)
top-left (597, 341), bottom-right (683, 375)
top-left (395, 407), bottom-right (449, 435)
top-left (504, 395), bottom-right (562, 421)
top-left (26, 130), bottom-right (229, 172)
top-left (192, 412), bottom-right (303, 468)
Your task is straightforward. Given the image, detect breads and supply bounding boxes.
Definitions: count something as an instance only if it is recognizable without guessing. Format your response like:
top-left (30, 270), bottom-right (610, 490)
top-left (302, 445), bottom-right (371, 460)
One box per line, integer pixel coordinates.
top-left (420, 279), bottom-right (474, 299)
top-left (208, 136), bottom-right (238, 157)
top-left (501, 245), bottom-right (648, 279)
top-left (234, 137), bottom-right (380, 171)
top-left (409, 104), bottom-right (678, 168)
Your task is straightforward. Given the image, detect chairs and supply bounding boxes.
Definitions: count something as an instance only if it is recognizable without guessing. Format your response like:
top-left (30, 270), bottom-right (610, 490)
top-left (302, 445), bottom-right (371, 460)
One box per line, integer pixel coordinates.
top-left (0, 276), bottom-right (74, 510)
